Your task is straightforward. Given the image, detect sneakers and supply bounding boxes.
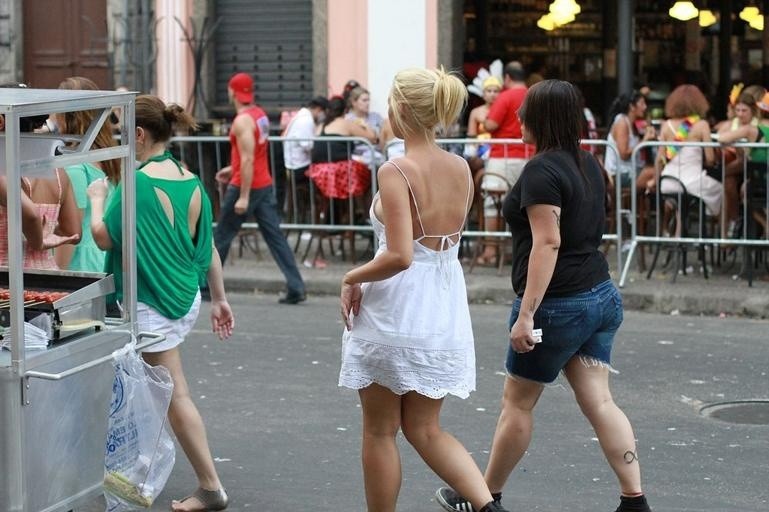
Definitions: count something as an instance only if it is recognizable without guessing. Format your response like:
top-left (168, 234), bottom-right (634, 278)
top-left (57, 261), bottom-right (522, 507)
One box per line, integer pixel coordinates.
top-left (435, 488), bottom-right (503, 512)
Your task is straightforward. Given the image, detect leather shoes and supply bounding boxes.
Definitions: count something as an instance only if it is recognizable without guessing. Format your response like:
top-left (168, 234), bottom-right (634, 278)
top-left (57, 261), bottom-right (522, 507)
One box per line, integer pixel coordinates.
top-left (279, 291), bottom-right (308, 303)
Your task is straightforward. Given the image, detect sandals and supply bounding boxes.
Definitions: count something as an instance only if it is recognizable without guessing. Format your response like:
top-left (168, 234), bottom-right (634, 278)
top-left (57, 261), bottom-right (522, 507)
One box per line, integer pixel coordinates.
top-left (172, 486), bottom-right (229, 511)
top-left (470, 256), bottom-right (497, 267)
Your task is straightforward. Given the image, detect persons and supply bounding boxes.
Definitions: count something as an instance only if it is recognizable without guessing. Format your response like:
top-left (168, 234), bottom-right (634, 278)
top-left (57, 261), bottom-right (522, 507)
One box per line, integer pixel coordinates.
top-left (0, 76), bottom-right (235, 512)
top-left (435, 80), bottom-right (650, 512)
top-left (338, 66), bottom-right (504, 512)
top-left (198, 61), bottom-right (768, 302)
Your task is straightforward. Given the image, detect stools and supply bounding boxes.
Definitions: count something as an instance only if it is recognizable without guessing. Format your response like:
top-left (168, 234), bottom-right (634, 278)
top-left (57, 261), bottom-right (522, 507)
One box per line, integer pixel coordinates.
top-left (217, 155), bottom-right (769, 283)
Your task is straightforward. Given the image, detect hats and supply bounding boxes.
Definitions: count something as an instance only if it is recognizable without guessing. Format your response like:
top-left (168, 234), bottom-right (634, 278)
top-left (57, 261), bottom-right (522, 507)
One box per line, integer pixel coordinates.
top-left (483, 77), bottom-right (501, 89)
top-left (228, 74), bottom-right (254, 103)
